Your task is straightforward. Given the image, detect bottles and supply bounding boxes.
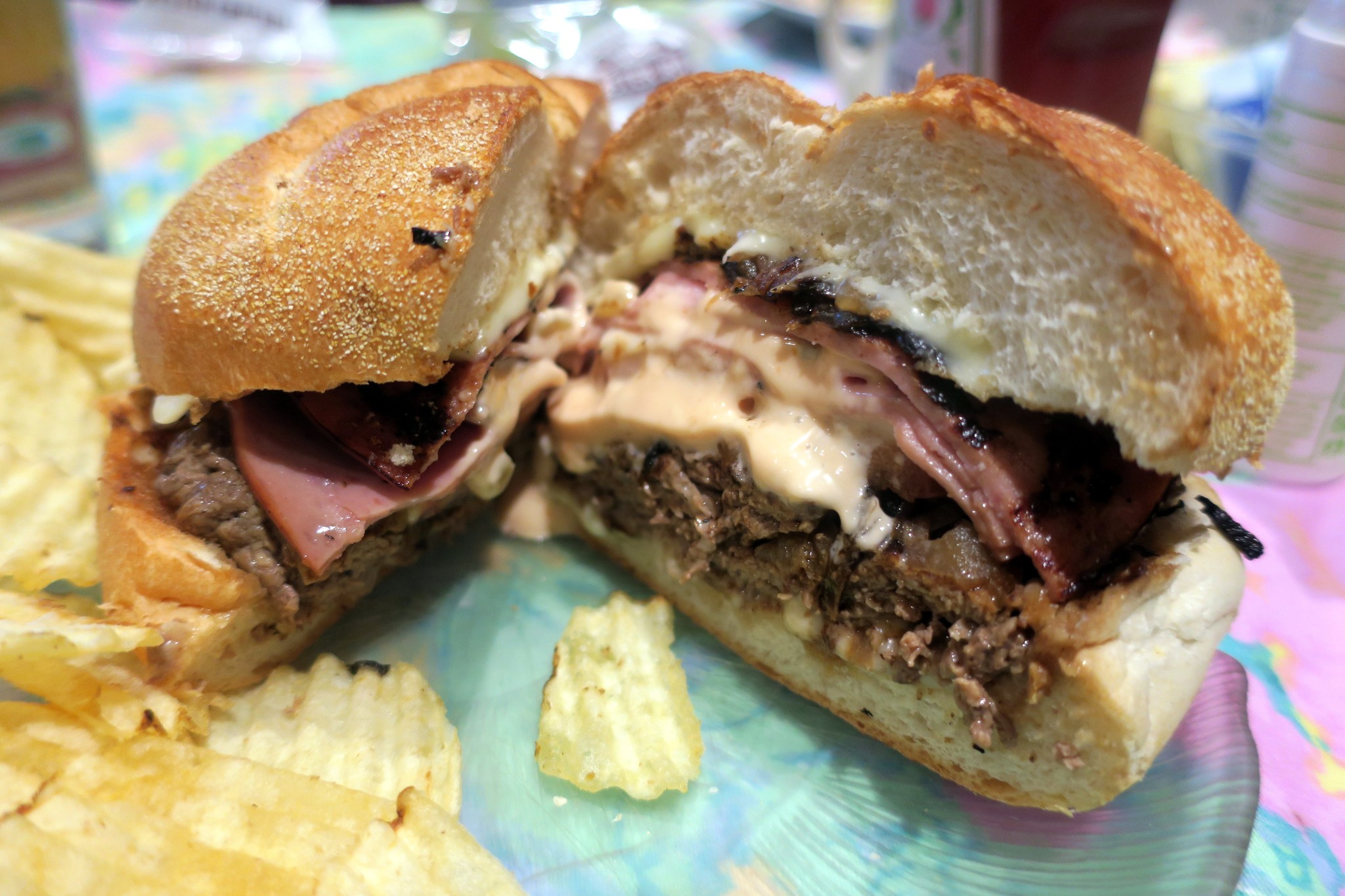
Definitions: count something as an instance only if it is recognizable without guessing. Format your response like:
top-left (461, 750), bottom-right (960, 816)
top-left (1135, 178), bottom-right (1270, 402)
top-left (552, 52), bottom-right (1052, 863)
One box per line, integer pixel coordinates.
top-left (1206, 1), bottom-right (1345, 489)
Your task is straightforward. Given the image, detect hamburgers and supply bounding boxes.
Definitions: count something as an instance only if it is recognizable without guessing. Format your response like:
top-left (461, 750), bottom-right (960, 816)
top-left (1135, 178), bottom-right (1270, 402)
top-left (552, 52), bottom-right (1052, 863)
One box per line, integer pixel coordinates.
top-left (98, 55), bottom-right (1295, 820)
